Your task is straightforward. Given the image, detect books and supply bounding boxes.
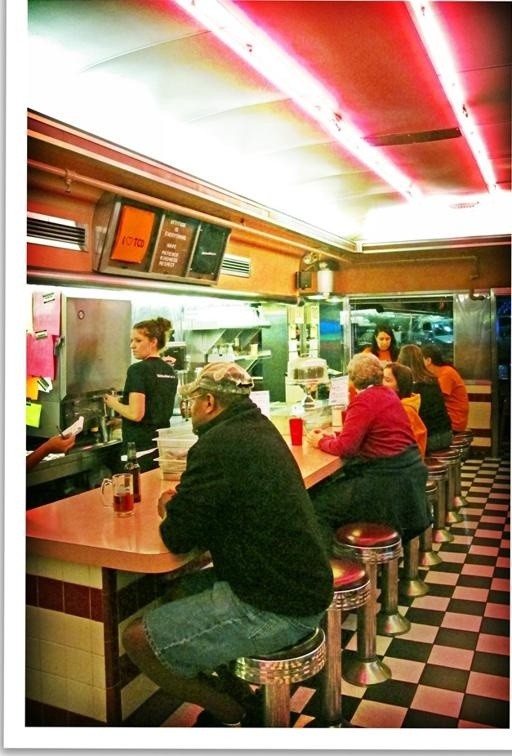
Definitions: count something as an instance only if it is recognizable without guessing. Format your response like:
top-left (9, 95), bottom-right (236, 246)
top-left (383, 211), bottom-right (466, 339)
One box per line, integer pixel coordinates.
top-left (58, 416), bottom-right (84, 437)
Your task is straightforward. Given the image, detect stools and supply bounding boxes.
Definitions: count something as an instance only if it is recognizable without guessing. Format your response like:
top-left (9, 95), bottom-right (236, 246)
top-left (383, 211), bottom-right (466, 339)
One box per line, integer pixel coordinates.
top-left (324, 557), bottom-right (372, 726)
top-left (378, 426), bottom-right (473, 635)
top-left (332, 521), bottom-right (401, 688)
top-left (228, 624), bottom-right (329, 728)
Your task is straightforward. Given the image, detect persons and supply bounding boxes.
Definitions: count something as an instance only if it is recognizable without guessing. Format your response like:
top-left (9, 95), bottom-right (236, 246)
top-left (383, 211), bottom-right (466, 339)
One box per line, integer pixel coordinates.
top-left (105, 316), bottom-right (177, 474)
top-left (27, 432), bottom-right (77, 470)
top-left (122, 362), bottom-right (333, 726)
top-left (306, 353), bottom-right (433, 559)
top-left (351, 325), bottom-right (469, 460)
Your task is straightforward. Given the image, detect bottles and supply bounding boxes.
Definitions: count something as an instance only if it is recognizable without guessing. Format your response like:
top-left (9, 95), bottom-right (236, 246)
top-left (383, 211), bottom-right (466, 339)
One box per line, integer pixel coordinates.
top-left (123, 442), bottom-right (141, 503)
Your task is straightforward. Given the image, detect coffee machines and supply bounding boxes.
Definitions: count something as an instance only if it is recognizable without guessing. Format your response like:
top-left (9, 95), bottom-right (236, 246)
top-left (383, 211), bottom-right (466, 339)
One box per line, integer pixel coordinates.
top-left (161, 339), bottom-right (190, 416)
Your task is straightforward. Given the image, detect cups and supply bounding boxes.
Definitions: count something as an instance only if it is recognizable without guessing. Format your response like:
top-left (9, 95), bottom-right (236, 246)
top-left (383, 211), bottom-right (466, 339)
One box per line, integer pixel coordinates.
top-left (99, 473), bottom-right (135, 517)
top-left (332, 410), bottom-right (342, 427)
top-left (250, 343), bottom-right (259, 355)
top-left (289, 418), bottom-right (303, 446)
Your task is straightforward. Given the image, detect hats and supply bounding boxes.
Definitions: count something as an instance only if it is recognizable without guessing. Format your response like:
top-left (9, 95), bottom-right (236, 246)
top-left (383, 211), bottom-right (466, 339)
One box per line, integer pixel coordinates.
top-left (177, 359), bottom-right (255, 398)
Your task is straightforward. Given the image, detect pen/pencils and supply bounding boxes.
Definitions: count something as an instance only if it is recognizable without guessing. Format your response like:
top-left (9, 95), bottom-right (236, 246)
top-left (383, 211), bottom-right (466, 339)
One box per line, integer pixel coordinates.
top-left (56, 425), bottom-right (66, 440)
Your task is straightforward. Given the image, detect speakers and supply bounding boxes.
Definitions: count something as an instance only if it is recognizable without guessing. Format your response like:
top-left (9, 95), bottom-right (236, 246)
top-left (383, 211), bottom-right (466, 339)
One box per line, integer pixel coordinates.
top-left (297, 271), bottom-right (311, 287)
